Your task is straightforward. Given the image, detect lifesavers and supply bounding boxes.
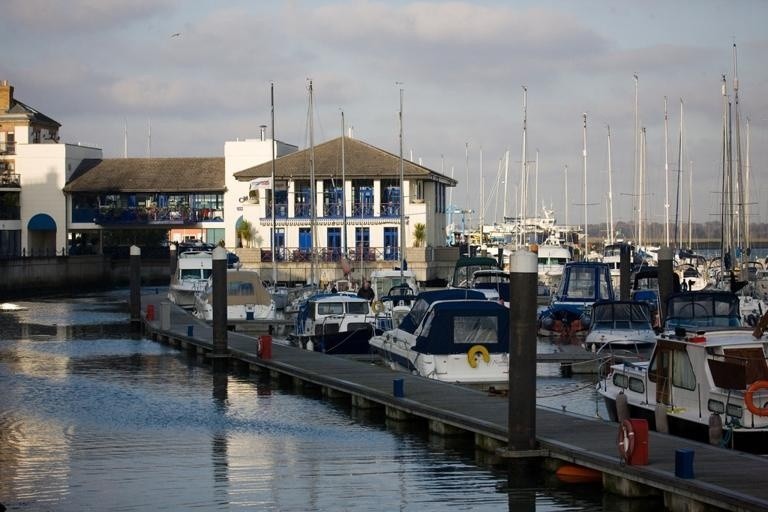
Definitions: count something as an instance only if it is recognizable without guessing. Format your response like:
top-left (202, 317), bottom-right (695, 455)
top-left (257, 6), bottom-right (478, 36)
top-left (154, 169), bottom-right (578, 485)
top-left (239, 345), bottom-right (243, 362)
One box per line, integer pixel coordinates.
top-left (557, 464), bottom-right (601, 483)
top-left (616, 420), bottom-right (635, 460)
top-left (372, 301), bottom-right (384, 313)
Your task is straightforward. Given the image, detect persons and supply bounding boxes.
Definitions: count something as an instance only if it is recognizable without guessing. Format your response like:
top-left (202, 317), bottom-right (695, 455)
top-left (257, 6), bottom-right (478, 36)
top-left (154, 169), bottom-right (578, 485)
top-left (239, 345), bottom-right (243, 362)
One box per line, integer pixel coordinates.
top-left (357, 281), bottom-right (375, 307)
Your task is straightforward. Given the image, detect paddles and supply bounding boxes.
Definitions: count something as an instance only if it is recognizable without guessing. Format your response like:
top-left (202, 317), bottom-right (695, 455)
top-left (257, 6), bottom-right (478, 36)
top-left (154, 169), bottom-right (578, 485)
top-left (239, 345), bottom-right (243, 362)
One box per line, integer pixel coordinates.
top-left (745, 380), bottom-right (768, 416)
top-left (468, 344), bottom-right (490, 368)
